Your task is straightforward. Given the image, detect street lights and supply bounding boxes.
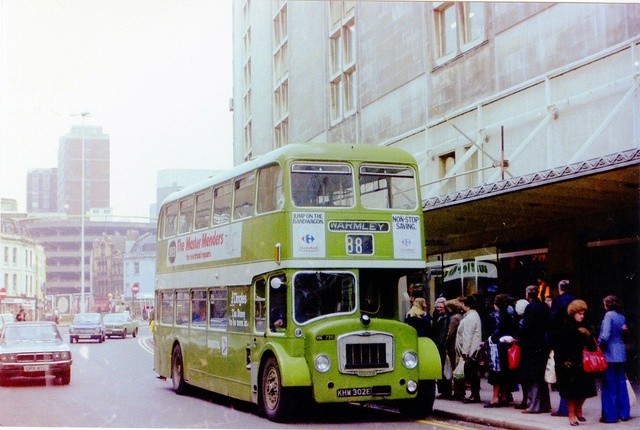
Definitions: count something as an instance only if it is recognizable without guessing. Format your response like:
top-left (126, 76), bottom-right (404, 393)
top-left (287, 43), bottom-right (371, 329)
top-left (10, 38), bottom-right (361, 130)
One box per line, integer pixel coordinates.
top-left (80, 112), bottom-right (90, 312)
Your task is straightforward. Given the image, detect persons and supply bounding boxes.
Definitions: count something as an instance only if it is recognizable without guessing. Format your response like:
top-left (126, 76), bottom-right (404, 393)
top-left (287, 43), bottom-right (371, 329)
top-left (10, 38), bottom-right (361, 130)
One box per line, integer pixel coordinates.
top-left (150, 307), bottom-right (156, 343)
top-left (434, 297), bottom-right (450, 400)
top-left (16, 310), bottom-right (26, 321)
top-left (521, 285), bottom-right (553, 413)
top-left (551, 281), bottom-right (581, 417)
top-left (597, 295), bottom-right (632, 423)
top-left (557, 299), bottom-right (598, 426)
top-left (457, 294), bottom-right (482, 404)
top-left (532, 271), bottom-right (551, 305)
top-left (446, 299), bottom-right (466, 401)
top-left (273, 282), bottom-right (312, 329)
top-left (484, 295), bottom-right (516, 407)
top-left (406, 298), bottom-right (433, 341)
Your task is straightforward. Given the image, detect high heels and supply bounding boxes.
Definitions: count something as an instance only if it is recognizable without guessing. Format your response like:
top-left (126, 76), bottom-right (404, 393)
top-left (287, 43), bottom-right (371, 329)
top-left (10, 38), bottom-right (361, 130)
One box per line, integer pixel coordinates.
top-left (499, 399), bottom-right (509, 407)
top-left (578, 417), bottom-right (586, 421)
top-left (570, 421), bottom-right (579, 426)
top-left (483, 400), bottom-right (500, 408)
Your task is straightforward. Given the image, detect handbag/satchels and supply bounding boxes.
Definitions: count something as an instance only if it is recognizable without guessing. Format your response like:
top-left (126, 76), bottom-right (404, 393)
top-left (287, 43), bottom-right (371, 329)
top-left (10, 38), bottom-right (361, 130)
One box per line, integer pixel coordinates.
top-left (583, 336), bottom-right (608, 372)
top-left (487, 336), bottom-right (501, 371)
top-left (508, 339), bottom-right (520, 368)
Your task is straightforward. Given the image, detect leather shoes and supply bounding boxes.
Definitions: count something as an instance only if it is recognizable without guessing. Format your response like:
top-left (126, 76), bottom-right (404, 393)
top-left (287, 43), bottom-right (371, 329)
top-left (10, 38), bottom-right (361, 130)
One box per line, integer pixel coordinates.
top-left (521, 409), bottom-right (533, 414)
top-left (551, 411), bottom-right (566, 416)
top-left (514, 401), bottom-right (528, 409)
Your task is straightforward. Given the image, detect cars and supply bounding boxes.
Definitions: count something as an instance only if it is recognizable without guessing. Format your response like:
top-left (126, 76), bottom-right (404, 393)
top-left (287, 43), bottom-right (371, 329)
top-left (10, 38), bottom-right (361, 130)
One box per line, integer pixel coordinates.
top-left (69, 312), bottom-right (105, 343)
top-left (104, 313), bottom-right (139, 339)
top-left (1, 320), bottom-right (73, 386)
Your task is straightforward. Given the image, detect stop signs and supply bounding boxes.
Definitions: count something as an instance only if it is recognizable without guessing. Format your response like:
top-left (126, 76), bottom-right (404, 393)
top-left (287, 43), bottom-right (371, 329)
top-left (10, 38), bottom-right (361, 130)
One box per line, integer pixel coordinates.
top-left (0, 288), bottom-right (7, 300)
top-left (132, 282), bottom-right (138, 294)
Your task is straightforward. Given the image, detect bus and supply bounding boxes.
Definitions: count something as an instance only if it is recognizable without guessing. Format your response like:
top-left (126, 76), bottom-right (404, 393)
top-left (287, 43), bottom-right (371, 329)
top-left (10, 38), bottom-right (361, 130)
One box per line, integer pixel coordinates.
top-left (155, 143), bottom-right (444, 423)
